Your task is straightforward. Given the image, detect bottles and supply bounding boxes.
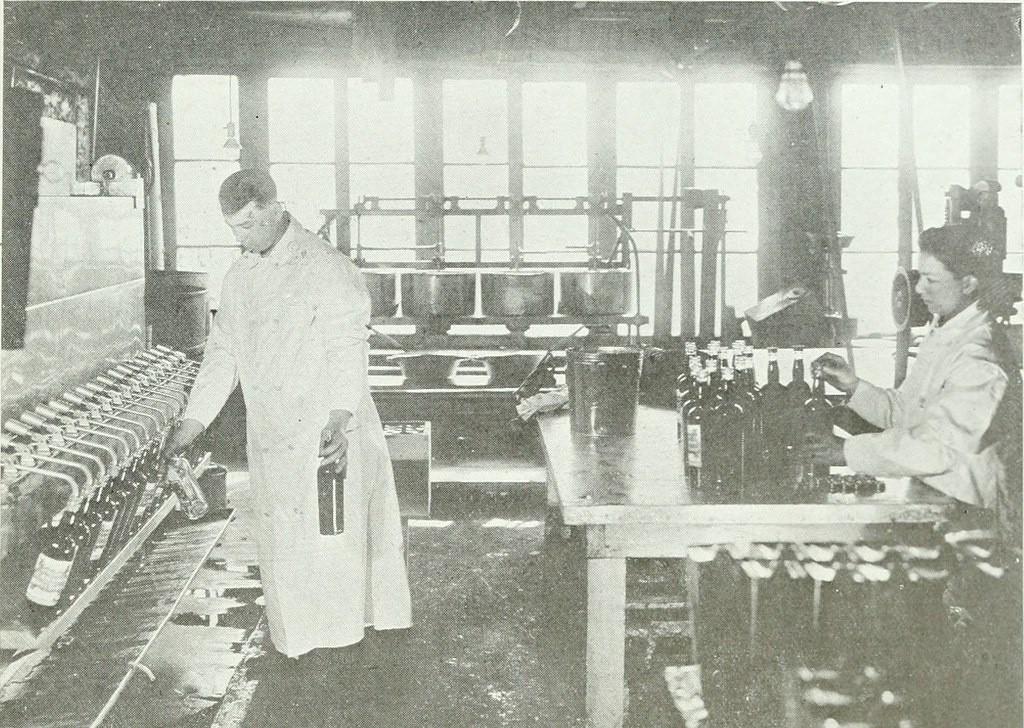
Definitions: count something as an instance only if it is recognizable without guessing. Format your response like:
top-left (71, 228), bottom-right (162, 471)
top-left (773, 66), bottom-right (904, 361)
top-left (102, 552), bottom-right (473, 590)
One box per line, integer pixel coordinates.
top-left (24, 434), bottom-right (209, 605)
top-left (678, 338), bottom-right (831, 501)
top-left (316, 429), bottom-right (346, 537)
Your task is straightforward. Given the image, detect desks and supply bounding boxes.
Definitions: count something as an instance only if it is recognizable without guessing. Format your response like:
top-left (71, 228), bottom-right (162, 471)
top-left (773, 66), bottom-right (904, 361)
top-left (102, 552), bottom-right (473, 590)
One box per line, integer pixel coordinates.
top-left (526, 366), bottom-right (1000, 728)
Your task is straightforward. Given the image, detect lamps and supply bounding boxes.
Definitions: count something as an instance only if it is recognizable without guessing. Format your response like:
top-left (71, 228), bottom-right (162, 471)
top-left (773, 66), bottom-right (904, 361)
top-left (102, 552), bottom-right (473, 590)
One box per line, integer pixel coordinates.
top-left (771, 57), bottom-right (817, 111)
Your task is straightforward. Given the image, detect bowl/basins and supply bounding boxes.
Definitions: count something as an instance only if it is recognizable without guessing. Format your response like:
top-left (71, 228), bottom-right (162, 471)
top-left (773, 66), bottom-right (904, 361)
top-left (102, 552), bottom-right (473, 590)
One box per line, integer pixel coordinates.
top-left (478, 269), bottom-right (555, 317)
top-left (400, 271), bottom-right (476, 317)
top-left (477, 353), bottom-right (558, 382)
top-left (360, 272), bottom-right (395, 317)
top-left (385, 351), bottom-right (468, 382)
top-left (558, 269), bottom-right (635, 316)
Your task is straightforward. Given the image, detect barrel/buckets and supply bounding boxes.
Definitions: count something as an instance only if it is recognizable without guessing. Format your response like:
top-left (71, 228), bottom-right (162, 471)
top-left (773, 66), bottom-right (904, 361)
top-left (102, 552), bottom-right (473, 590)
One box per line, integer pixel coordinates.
top-left (152, 285), bottom-right (207, 356)
top-left (145, 270), bottom-right (211, 341)
top-left (565, 345), bottom-right (644, 437)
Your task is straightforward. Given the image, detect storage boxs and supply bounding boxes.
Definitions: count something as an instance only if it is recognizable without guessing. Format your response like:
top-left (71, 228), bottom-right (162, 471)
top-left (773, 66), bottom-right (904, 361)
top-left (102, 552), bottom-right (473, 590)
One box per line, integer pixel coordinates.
top-left (383, 420), bottom-right (433, 516)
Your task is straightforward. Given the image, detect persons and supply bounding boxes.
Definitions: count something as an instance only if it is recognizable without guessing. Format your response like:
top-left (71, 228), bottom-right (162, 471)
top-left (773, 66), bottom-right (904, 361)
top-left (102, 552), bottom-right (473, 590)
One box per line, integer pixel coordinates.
top-left (163, 169), bottom-right (415, 657)
top-left (812, 224), bottom-right (1022, 514)
